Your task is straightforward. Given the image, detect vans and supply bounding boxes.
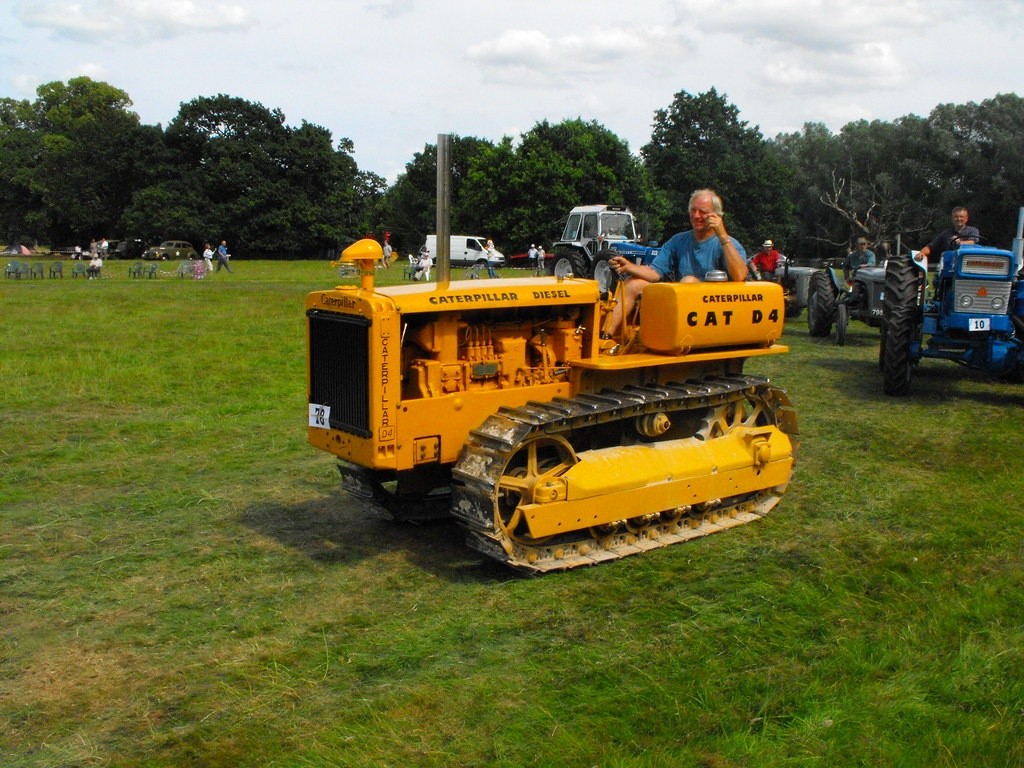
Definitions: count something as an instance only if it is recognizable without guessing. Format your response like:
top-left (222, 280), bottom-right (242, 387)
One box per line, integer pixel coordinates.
top-left (425, 234), bottom-right (506, 267)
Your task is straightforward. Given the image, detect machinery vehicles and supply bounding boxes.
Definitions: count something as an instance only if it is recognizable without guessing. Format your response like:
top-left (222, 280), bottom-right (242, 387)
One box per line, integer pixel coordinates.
top-left (302, 133), bottom-right (803, 575)
top-left (877, 206), bottom-right (1024, 397)
top-left (544, 202), bottom-right (886, 348)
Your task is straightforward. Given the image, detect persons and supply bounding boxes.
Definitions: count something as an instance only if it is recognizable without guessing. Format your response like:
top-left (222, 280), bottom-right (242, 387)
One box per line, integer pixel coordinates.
top-left (600, 189), bottom-right (749, 341)
top-left (86, 254), bottom-right (103, 280)
top-left (751, 240), bottom-right (781, 280)
top-left (914, 207), bottom-right (979, 298)
top-left (485, 239), bottom-right (499, 279)
top-left (378, 241), bottom-right (392, 270)
top-left (842, 238), bottom-right (876, 286)
top-left (71, 238), bottom-right (109, 260)
top-left (203, 241), bottom-right (216, 276)
top-left (412, 253), bottom-right (433, 281)
top-left (527, 243), bottom-right (546, 270)
top-left (217, 241), bottom-right (234, 273)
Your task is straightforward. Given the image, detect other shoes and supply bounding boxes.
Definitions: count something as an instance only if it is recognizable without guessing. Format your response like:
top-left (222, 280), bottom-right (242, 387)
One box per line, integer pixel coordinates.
top-left (89, 276), bottom-right (97, 280)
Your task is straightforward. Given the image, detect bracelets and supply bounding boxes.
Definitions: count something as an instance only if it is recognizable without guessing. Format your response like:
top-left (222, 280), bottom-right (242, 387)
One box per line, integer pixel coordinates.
top-left (721, 239), bottom-right (731, 246)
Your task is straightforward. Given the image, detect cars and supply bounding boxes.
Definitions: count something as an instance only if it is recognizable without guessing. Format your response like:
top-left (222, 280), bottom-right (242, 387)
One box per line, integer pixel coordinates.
top-left (746, 250), bottom-right (791, 265)
top-left (142, 240), bottom-right (201, 261)
top-left (93, 239), bottom-right (119, 260)
top-left (116, 241), bottom-right (147, 259)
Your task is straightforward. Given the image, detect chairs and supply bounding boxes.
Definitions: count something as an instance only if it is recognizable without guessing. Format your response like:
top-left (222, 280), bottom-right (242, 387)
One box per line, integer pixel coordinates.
top-left (191, 259), bottom-right (206, 279)
top-left (128, 262), bottom-right (143, 279)
top-left (50, 262), bottom-right (63, 278)
top-left (142, 262), bottom-right (158, 278)
top-left (72, 262), bottom-right (85, 279)
top-left (403, 266), bottom-right (416, 280)
top-left (4, 260), bottom-right (45, 278)
top-left (86, 269), bottom-right (101, 277)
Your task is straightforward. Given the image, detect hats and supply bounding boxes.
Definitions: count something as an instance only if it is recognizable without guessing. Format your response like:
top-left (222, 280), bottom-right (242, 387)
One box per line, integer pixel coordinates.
top-left (93, 253), bottom-right (98, 258)
top-left (762, 239), bottom-right (774, 247)
top-left (538, 246), bottom-right (542, 249)
top-left (531, 244), bottom-right (535, 247)
top-left (857, 237), bottom-right (867, 244)
